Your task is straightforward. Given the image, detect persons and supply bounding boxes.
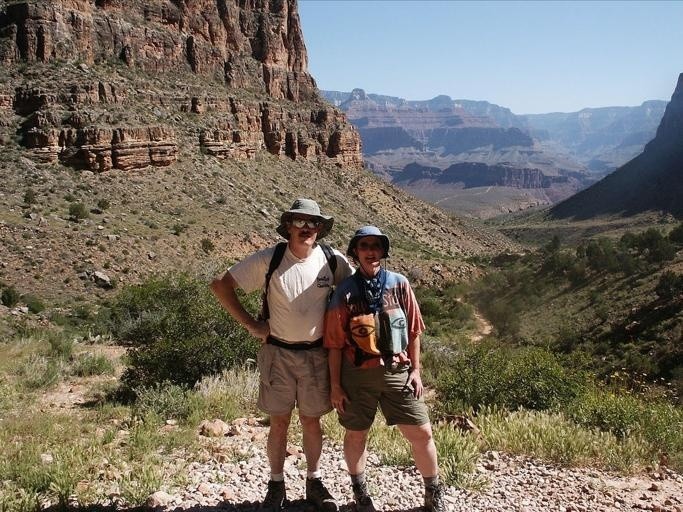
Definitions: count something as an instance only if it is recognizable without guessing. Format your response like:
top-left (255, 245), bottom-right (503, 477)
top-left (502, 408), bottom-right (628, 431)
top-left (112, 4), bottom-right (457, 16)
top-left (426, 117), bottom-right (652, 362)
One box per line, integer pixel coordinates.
top-left (322, 225), bottom-right (447, 512)
top-left (208, 195), bottom-right (357, 512)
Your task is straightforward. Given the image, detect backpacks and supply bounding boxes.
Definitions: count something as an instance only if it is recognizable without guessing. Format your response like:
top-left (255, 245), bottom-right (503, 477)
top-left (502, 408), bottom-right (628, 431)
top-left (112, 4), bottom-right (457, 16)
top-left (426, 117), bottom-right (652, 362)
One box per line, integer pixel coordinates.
top-left (259, 242), bottom-right (336, 323)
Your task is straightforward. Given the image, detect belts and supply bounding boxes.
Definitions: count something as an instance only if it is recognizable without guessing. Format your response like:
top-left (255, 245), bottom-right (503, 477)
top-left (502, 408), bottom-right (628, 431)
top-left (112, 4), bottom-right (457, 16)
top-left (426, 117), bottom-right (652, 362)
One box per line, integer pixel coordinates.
top-left (263, 335), bottom-right (323, 350)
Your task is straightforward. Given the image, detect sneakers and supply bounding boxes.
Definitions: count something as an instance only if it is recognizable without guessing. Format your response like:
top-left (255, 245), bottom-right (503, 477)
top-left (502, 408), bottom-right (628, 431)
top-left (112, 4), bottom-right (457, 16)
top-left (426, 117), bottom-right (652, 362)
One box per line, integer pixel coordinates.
top-left (305, 478), bottom-right (340, 509)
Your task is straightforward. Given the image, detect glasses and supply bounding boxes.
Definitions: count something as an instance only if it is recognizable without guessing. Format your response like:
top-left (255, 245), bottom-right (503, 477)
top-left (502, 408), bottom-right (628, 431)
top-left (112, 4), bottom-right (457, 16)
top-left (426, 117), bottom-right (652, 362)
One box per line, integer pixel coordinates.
top-left (354, 242), bottom-right (382, 251)
top-left (284, 217), bottom-right (322, 231)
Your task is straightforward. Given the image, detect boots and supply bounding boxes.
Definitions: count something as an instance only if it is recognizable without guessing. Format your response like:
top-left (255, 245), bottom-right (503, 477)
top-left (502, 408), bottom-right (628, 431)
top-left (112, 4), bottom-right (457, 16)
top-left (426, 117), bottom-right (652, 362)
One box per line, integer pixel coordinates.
top-left (424, 486), bottom-right (448, 512)
top-left (262, 479), bottom-right (291, 511)
top-left (351, 482), bottom-right (378, 509)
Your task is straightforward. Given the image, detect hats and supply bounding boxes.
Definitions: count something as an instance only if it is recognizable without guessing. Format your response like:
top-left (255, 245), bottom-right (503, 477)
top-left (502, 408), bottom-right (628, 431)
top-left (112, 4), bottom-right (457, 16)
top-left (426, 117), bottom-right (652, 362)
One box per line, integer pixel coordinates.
top-left (274, 196), bottom-right (334, 243)
top-left (346, 224), bottom-right (392, 262)
